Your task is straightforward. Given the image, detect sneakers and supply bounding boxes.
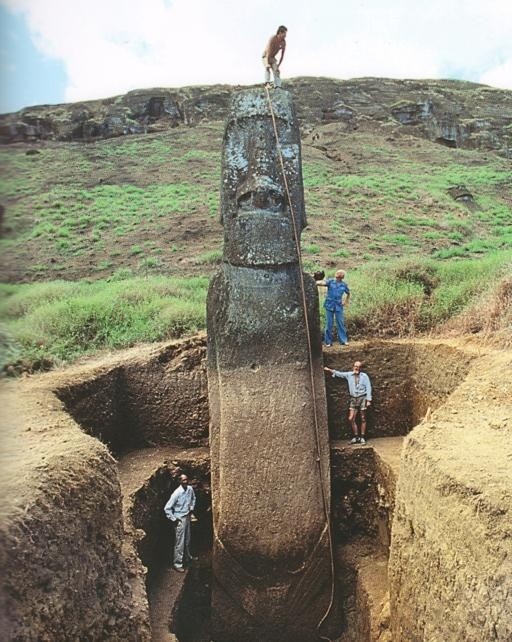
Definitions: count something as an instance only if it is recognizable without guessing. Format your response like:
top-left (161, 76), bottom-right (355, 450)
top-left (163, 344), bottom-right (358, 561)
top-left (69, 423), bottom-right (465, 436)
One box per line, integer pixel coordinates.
top-left (344, 341), bottom-right (349, 346)
top-left (326, 343), bottom-right (332, 347)
top-left (350, 436), bottom-right (360, 443)
top-left (360, 437), bottom-right (365, 446)
top-left (175, 567), bottom-right (185, 572)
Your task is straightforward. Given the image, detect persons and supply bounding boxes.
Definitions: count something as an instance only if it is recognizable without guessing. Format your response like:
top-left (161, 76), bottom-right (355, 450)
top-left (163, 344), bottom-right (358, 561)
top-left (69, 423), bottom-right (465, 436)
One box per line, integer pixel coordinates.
top-left (323, 361), bottom-right (374, 445)
top-left (163, 473), bottom-right (199, 573)
top-left (315, 269), bottom-right (351, 347)
top-left (261, 25), bottom-right (287, 87)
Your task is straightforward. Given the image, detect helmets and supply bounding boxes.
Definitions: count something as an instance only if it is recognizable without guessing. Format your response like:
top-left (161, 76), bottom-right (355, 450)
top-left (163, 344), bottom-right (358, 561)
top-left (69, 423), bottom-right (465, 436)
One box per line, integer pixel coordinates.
top-left (336, 270), bottom-right (345, 278)
